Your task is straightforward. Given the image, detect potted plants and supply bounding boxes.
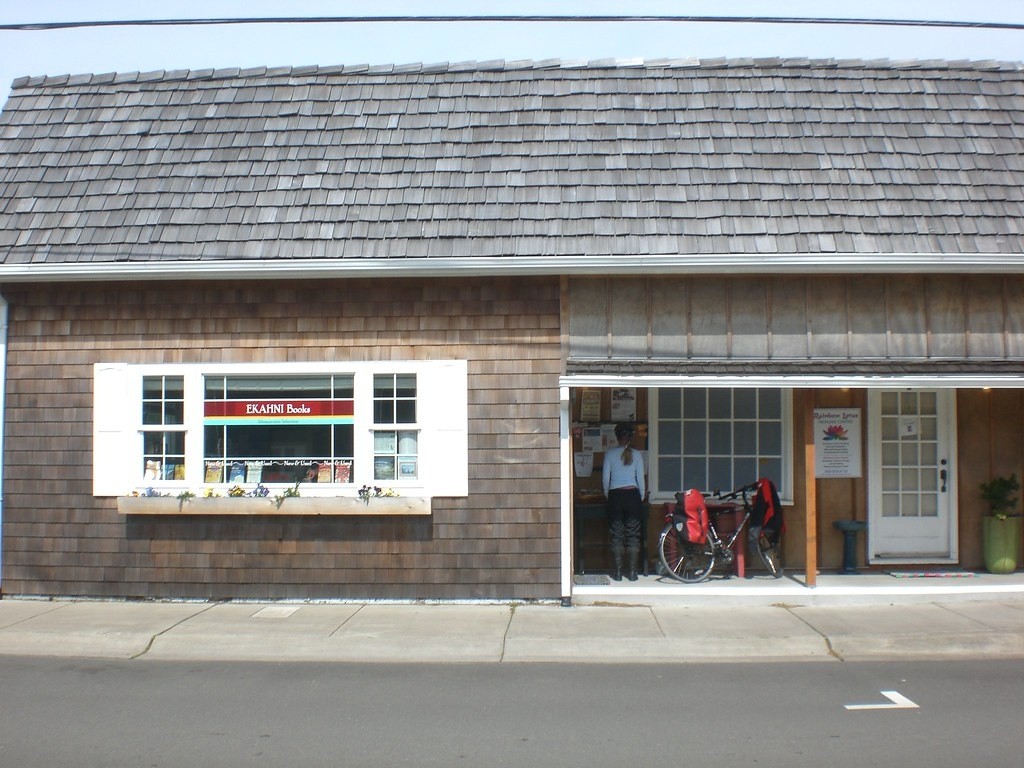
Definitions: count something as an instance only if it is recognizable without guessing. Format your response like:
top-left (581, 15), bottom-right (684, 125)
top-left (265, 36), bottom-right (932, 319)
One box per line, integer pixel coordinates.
top-left (980, 473), bottom-right (1022, 573)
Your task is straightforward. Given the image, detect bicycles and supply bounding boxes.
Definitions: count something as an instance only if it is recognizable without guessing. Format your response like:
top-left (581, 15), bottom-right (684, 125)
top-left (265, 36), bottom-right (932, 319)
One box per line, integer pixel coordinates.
top-left (657, 477), bottom-right (785, 583)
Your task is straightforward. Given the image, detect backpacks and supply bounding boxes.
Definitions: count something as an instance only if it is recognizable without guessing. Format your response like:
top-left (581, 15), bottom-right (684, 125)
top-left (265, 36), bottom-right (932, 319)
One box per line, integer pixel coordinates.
top-left (673, 489), bottom-right (708, 545)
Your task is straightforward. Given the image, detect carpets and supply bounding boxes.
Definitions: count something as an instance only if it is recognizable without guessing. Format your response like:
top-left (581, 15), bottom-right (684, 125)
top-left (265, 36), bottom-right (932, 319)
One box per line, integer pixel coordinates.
top-left (882, 567), bottom-right (977, 577)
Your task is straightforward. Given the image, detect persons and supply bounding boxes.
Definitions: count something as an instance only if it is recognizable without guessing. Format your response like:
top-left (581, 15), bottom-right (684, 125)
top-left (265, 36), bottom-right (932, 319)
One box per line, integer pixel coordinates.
top-left (603, 423), bottom-right (647, 581)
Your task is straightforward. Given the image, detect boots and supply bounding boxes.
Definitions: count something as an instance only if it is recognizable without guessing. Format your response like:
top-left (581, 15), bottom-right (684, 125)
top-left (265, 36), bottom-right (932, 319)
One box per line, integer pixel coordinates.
top-left (610, 547), bottom-right (624, 581)
top-left (625, 547), bottom-right (639, 582)
